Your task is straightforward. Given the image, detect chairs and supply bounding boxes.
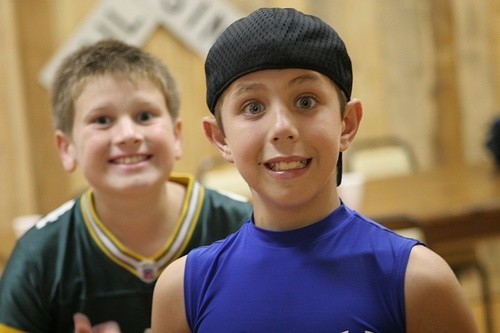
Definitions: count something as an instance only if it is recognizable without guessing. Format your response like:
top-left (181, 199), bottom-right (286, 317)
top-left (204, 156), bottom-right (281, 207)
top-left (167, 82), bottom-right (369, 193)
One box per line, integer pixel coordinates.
top-left (348, 141), bottom-right (492, 333)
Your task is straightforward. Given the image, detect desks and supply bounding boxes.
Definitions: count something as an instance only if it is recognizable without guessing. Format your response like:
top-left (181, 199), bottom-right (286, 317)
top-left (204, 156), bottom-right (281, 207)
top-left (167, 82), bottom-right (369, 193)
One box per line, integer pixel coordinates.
top-left (359, 159), bottom-right (498, 228)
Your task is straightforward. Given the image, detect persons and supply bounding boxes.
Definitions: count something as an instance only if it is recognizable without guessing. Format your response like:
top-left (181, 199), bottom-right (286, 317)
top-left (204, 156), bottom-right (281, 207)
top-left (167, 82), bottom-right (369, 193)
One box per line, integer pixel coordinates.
top-left (0, 37), bottom-right (254, 333)
top-left (147, 7), bottom-right (482, 333)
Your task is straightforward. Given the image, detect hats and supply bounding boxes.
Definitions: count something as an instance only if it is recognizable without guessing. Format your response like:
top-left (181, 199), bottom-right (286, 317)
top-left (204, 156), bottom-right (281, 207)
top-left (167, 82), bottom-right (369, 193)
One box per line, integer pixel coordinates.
top-left (203, 6), bottom-right (353, 189)
